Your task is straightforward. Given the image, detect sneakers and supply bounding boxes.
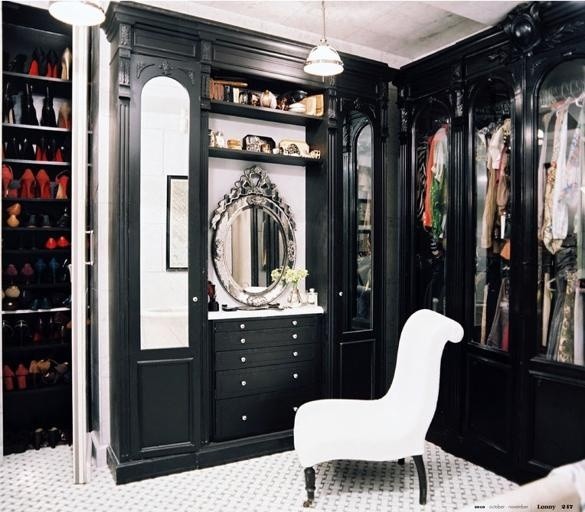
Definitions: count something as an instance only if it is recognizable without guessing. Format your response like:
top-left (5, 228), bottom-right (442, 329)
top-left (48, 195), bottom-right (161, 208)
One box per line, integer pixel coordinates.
top-left (6, 215), bottom-right (20, 228)
top-left (7, 202), bottom-right (21, 217)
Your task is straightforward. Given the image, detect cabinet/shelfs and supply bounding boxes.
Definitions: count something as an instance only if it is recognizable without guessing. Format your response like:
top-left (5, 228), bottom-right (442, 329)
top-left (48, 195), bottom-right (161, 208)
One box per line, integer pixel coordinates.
top-left (99, 0), bottom-right (391, 486)
top-left (1, 0), bottom-right (96, 455)
top-left (396, 0), bottom-right (585, 470)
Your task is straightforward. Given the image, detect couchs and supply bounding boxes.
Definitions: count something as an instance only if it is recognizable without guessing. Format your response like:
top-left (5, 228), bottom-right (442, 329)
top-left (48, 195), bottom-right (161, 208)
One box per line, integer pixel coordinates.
top-left (293, 307), bottom-right (465, 506)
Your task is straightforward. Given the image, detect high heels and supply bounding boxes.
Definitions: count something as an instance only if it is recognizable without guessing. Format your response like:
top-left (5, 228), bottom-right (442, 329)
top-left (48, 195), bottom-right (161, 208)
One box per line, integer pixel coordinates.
top-left (35, 168), bottom-right (52, 199)
top-left (49, 135), bottom-right (64, 161)
top-left (6, 54), bottom-right (27, 73)
top-left (2, 164), bottom-right (17, 198)
top-left (20, 262), bottom-right (34, 285)
top-left (3, 365), bottom-right (15, 391)
top-left (16, 364), bottom-right (28, 389)
top-left (35, 131), bottom-right (47, 161)
top-left (3, 78), bottom-right (15, 123)
top-left (54, 207), bottom-right (69, 227)
top-left (27, 47), bottom-right (43, 76)
top-left (29, 360), bottom-right (44, 388)
top-left (41, 82), bottom-right (56, 124)
top-left (55, 169), bottom-right (69, 199)
top-left (6, 133), bottom-right (19, 158)
top-left (57, 102), bottom-right (71, 128)
top-left (50, 258), bottom-right (69, 287)
top-left (20, 168), bottom-right (35, 199)
top-left (6, 264), bottom-right (18, 284)
top-left (21, 80), bottom-right (37, 124)
top-left (2, 320), bottom-right (14, 352)
top-left (61, 47), bottom-right (71, 80)
top-left (35, 258), bottom-right (47, 284)
top-left (45, 50), bottom-right (58, 78)
top-left (34, 317), bottom-right (48, 345)
top-left (21, 132), bottom-right (35, 159)
top-left (47, 311), bottom-right (65, 344)
top-left (12, 319), bottom-right (29, 349)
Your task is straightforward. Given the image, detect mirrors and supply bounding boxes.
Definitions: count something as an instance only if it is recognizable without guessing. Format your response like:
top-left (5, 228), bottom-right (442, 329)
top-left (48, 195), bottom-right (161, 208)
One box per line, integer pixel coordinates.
top-left (138, 71), bottom-right (189, 351)
top-left (210, 164), bottom-right (298, 307)
top-left (349, 116), bottom-right (379, 330)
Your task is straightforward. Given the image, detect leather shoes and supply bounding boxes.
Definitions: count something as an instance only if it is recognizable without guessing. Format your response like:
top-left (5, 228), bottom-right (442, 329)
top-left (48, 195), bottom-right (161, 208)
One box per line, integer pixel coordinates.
top-left (42, 236), bottom-right (58, 249)
top-left (47, 426), bottom-right (59, 449)
top-left (34, 428), bottom-right (45, 450)
top-left (27, 214), bottom-right (38, 228)
top-left (56, 235), bottom-right (69, 249)
top-left (41, 214), bottom-right (52, 228)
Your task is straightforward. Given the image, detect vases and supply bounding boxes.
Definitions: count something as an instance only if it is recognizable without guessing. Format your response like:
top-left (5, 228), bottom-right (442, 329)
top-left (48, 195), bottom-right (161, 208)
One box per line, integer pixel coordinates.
top-left (287, 284), bottom-right (304, 308)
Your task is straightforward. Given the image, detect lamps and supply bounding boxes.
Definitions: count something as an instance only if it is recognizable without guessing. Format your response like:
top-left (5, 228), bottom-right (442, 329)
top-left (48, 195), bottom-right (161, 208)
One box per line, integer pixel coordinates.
top-left (45, 0), bottom-right (108, 28)
top-left (301, 1), bottom-right (346, 78)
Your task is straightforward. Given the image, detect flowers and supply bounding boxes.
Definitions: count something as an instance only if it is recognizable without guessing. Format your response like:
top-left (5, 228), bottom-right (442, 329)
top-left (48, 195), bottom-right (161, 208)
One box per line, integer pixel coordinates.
top-left (271, 266), bottom-right (309, 302)
